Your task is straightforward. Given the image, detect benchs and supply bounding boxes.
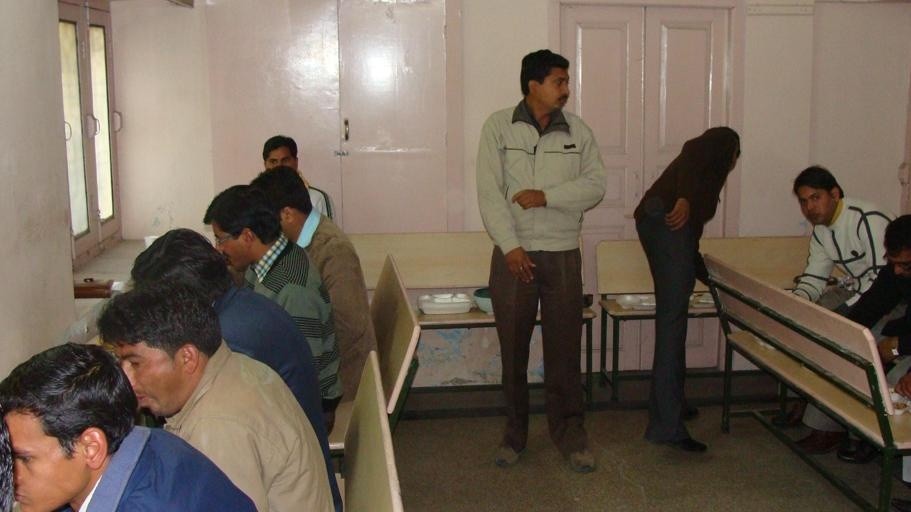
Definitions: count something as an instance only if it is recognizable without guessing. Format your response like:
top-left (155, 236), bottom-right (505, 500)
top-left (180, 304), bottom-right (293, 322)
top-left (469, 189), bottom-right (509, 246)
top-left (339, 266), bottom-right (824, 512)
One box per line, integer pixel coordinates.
top-left (699, 251), bottom-right (910, 511)
top-left (596, 233), bottom-right (839, 408)
top-left (327, 250), bottom-right (423, 469)
top-left (332, 349), bottom-right (404, 511)
top-left (342, 233), bottom-right (596, 418)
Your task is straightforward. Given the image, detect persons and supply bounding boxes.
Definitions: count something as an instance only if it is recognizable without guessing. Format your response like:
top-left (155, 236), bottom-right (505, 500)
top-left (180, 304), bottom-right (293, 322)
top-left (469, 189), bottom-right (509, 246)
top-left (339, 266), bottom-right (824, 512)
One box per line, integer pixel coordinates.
top-left (479, 50), bottom-right (599, 474)
top-left (246, 168), bottom-right (377, 400)
top-left (1, 342), bottom-right (267, 512)
top-left (0, 418), bottom-right (17, 512)
top-left (129, 229), bottom-right (345, 512)
top-left (248, 136), bottom-right (333, 248)
top-left (95, 281), bottom-right (345, 511)
top-left (634, 127), bottom-right (740, 455)
top-left (205, 187), bottom-right (340, 441)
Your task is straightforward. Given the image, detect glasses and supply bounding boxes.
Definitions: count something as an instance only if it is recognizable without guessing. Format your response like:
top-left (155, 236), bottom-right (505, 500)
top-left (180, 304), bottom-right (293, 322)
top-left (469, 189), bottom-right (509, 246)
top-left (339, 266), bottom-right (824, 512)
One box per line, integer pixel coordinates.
top-left (215, 233), bottom-right (240, 246)
top-left (882, 251), bottom-right (911, 271)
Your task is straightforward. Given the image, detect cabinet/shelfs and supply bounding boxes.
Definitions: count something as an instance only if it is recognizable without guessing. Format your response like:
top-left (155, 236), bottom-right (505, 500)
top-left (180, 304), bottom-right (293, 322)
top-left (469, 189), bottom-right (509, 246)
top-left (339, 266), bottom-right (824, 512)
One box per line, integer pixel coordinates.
top-left (204, 0), bottom-right (464, 238)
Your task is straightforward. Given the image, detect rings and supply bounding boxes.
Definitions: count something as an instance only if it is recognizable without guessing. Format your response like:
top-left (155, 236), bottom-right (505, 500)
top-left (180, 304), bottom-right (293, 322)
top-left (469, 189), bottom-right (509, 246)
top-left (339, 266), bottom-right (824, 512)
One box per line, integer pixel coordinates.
top-left (519, 266), bottom-right (523, 271)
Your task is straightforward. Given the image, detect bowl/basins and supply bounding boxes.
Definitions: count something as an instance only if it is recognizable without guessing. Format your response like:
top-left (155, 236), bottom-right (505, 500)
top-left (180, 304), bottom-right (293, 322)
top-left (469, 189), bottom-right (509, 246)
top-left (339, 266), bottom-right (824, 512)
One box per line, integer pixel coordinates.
top-left (472, 287), bottom-right (497, 314)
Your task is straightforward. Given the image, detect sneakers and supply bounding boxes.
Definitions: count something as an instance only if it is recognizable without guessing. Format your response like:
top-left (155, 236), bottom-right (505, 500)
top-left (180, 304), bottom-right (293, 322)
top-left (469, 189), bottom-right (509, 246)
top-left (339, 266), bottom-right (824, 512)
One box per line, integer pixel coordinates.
top-left (494, 433), bottom-right (530, 468)
top-left (788, 429), bottom-right (839, 457)
top-left (837, 435), bottom-right (881, 466)
top-left (772, 398), bottom-right (808, 427)
top-left (644, 425), bottom-right (710, 455)
top-left (565, 440), bottom-right (601, 474)
top-left (679, 399), bottom-right (703, 423)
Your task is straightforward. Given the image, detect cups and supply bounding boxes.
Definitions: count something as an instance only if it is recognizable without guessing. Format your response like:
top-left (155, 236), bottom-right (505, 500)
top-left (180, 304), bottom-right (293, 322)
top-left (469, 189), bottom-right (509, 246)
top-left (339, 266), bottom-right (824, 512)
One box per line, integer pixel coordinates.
top-left (144, 234), bottom-right (160, 251)
